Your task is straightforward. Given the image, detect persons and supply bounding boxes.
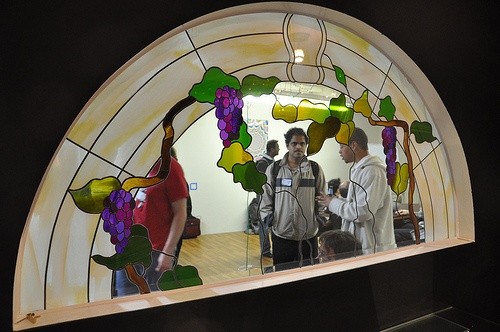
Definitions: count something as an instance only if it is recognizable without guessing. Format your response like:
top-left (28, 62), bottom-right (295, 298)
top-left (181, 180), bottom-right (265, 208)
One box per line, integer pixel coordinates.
top-left (257, 127), bottom-right (328, 265)
top-left (313, 126), bottom-right (398, 255)
top-left (255, 137), bottom-right (281, 258)
top-left (314, 226), bottom-right (364, 263)
top-left (115, 154), bottom-right (190, 296)
